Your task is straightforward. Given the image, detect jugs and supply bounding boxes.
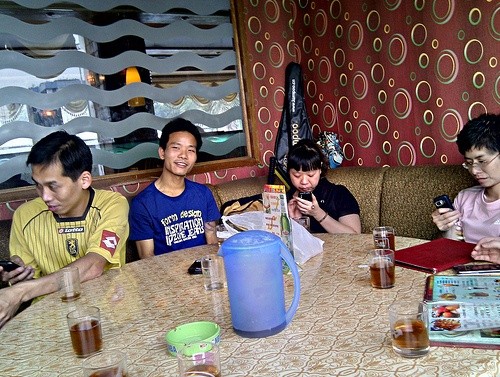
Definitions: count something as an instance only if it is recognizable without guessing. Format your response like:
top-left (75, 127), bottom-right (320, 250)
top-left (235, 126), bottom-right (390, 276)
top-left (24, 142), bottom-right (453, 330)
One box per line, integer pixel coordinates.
top-left (216, 230), bottom-right (301, 339)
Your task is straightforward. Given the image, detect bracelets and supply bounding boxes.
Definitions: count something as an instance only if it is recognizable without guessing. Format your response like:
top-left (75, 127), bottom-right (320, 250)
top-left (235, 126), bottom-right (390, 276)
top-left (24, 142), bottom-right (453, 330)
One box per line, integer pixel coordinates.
top-left (319, 213), bottom-right (328, 223)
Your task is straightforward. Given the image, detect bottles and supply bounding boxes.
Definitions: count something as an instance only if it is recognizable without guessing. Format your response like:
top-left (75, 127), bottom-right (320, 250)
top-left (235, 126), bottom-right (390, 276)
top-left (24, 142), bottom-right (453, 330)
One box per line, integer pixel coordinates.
top-left (279, 195), bottom-right (291, 275)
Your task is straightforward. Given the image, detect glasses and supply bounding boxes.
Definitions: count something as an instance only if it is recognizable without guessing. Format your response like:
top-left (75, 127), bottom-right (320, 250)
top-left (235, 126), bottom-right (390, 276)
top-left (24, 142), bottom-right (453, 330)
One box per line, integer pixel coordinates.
top-left (462, 152), bottom-right (500, 172)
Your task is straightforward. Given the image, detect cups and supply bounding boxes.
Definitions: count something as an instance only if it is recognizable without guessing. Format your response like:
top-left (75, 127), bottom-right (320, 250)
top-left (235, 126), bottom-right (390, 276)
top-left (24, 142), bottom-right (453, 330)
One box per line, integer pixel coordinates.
top-left (372, 225), bottom-right (396, 257)
top-left (82, 350), bottom-right (128, 377)
top-left (292, 216), bottom-right (311, 232)
top-left (367, 248), bottom-right (395, 289)
top-left (388, 300), bottom-right (431, 359)
top-left (54, 266), bottom-right (82, 303)
top-left (199, 253), bottom-right (225, 292)
top-left (66, 305), bottom-right (103, 358)
top-left (215, 223), bottom-right (233, 248)
top-left (176, 341), bottom-right (222, 377)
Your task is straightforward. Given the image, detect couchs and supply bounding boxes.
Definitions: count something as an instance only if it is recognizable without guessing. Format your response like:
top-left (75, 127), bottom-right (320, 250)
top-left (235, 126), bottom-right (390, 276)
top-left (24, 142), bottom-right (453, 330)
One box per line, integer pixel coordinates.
top-left (0, 166), bottom-right (482, 315)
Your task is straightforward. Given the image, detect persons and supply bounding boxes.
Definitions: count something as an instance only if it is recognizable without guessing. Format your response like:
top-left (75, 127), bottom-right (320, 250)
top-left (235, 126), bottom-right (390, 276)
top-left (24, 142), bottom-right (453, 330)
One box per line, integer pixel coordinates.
top-left (470, 237), bottom-right (500, 264)
top-left (0, 130), bottom-right (131, 328)
top-left (286, 140), bottom-right (361, 234)
top-left (431, 113), bottom-right (500, 243)
top-left (127, 117), bottom-right (221, 260)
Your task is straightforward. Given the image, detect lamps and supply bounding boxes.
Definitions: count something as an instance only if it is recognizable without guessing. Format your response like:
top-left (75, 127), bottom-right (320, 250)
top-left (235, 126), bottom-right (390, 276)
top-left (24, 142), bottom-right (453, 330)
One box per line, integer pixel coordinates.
top-left (126, 67), bottom-right (145, 107)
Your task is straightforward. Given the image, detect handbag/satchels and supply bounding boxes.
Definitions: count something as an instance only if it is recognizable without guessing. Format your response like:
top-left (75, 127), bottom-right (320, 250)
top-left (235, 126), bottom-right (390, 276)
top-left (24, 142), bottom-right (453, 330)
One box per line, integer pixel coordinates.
top-left (316, 131), bottom-right (345, 169)
top-left (220, 212), bottom-right (325, 266)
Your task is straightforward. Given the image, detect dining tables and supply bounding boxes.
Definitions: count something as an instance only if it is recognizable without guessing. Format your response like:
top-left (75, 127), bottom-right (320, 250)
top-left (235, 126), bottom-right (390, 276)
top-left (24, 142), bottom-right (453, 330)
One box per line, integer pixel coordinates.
top-left (0, 233), bottom-right (500, 377)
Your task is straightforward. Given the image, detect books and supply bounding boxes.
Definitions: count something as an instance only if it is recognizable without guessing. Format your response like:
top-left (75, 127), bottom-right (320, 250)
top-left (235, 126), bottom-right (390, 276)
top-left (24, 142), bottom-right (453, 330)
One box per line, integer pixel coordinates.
top-left (392, 238), bottom-right (480, 275)
top-left (418, 274), bottom-right (500, 349)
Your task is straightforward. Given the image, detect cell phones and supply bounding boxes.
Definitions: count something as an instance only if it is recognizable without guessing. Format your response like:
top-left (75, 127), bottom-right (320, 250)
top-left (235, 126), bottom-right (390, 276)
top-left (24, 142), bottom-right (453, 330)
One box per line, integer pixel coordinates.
top-left (299, 191), bottom-right (312, 203)
top-left (434, 194), bottom-right (454, 215)
top-left (0, 260), bottom-right (22, 278)
top-left (188, 259), bottom-right (211, 275)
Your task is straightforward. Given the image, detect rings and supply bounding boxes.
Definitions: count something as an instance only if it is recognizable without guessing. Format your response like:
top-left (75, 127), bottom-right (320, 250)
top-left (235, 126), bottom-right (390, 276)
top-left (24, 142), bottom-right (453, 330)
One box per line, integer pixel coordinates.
top-left (311, 205), bottom-right (315, 209)
